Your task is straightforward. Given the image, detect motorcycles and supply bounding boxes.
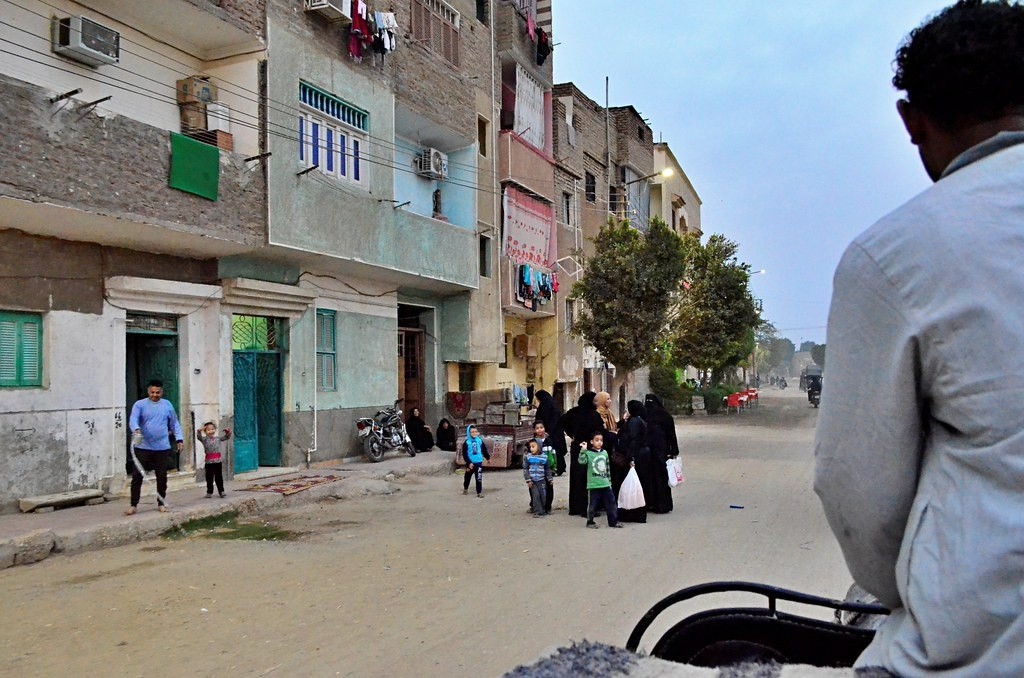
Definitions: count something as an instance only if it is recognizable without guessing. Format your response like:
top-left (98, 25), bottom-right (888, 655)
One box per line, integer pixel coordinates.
top-left (803, 374), bottom-right (824, 408)
top-left (355, 397), bottom-right (416, 463)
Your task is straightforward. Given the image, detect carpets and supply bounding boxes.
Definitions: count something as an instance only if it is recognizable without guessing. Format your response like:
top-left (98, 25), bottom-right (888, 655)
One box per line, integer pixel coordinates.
top-left (235, 474), bottom-right (350, 497)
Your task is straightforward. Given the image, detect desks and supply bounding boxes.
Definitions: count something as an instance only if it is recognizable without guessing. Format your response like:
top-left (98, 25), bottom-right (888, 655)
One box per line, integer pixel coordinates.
top-left (187, 129), bottom-right (233, 151)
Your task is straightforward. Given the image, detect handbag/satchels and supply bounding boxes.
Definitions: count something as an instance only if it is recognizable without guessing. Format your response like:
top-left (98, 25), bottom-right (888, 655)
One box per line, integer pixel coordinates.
top-left (665, 456), bottom-right (684, 488)
top-left (610, 451), bottom-right (628, 468)
top-left (617, 467), bottom-right (645, 510)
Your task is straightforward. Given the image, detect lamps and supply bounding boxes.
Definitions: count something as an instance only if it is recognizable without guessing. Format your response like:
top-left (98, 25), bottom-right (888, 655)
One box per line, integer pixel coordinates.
top-left (628, 169), bottom-right (674, 187)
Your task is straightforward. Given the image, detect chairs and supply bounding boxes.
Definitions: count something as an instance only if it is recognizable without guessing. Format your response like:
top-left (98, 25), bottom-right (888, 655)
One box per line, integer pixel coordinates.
top-left (722, 388), bottom-right (758, 414)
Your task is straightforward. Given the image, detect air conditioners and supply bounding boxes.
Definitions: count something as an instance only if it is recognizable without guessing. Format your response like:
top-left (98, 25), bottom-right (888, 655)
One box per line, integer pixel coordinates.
top-left (53, 15), bottom-right (121, 68)
top-left (310, 0), bottom-right (351, 20)
top-left (422, 148), bottom-right (448, 178)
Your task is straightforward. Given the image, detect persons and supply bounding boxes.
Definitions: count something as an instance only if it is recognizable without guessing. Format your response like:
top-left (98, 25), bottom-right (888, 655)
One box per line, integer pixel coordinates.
top-left (535, 389), bottom-right (568, 476)
top-left (197, 422), bottom-right (232, 498)
top-left (808, 377), bottom-right (822, 401)
top-left (780, 377), bottom-right (784, 385)
top-left (406, 408), bottom-right (435, 451)
top-left (559, 391), bottom-right (627, 518)
top-left (680, 378), bottom-right (700, 391)
top-left (462, 424), bottom-right (491, 498)
top-left (617, 400), bottom-right (650, 523)
top-left (577, 430), bottom-right (625, 529)
top-left (436, 418), bottom-right (456, 452)
top-left (524, 419), bottom-right (557, 517)
top-left (776, 376), bottom-right (779, 385)
top-left (812, 0), bottom-right (1024, 678)
top-left (645, 393), bottom-right (679, 514)
top-left (124, 379), bottom-right (184, 515)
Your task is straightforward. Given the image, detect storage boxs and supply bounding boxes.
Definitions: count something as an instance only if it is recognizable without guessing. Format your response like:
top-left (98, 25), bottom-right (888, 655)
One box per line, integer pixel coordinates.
top-left (176, 77), bottom-right (218, 105)
top-left (179, 103), bottom-right (208, 130)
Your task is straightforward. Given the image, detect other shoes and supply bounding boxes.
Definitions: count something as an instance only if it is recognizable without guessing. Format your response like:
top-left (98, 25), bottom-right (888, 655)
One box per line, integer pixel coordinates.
top-left (533, 513), bottom-right (539, 518)
top-left (545, 510), bottom-right (554, 515)
top-left (124, 507), bottom-right (137, 515)
top-left (205, 493), bottom-right (212, 498)
top-left (220, 492), bottom-right (227, 498)
top-left (580, 510), bottom-right (600, 517)
top-left (158, 505), bottom-right (167, 512)
top-left (477, 493), bottom-right (484, 498)
top-left (463, 489), bottom-right (468, 495)
top-left (527, 508), bottom-right (533, 513)
top-left (610, 521), bottom-right (625, 528)
top-left (420, 448), bottom-right (432, 452)
top-left (586, 522), bottom-right (598, 530)
top-left (414, 449), bottom-right (421, 453)
